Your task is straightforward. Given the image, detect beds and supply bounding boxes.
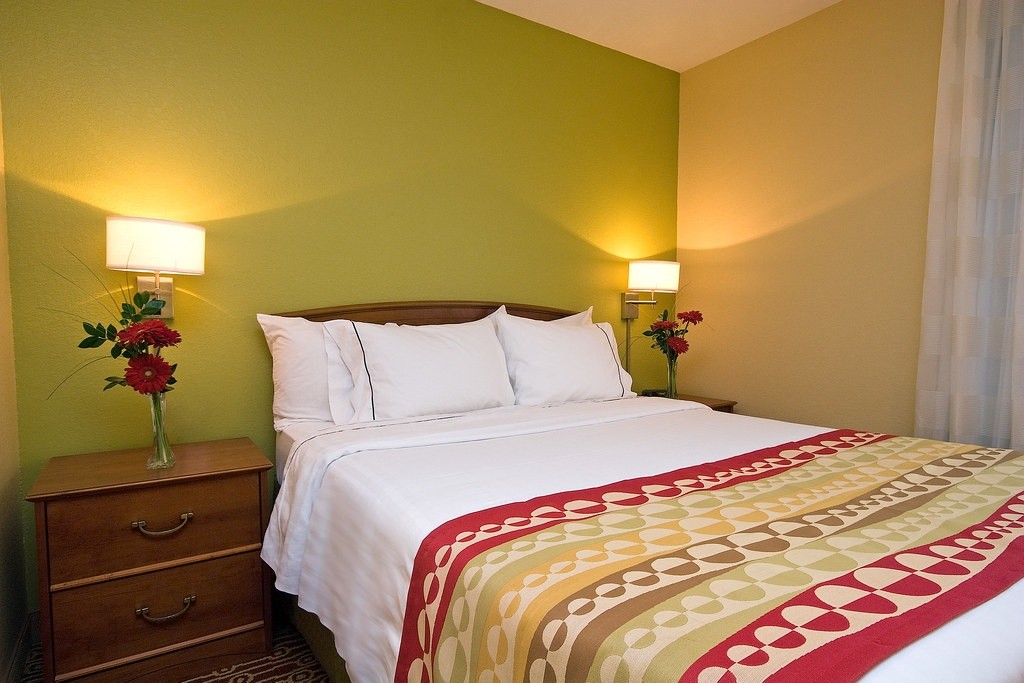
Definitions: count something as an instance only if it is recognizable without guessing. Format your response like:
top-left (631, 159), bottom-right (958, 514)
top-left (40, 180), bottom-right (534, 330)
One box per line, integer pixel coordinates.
top-left (259, 299), bottom-right (1024, 683)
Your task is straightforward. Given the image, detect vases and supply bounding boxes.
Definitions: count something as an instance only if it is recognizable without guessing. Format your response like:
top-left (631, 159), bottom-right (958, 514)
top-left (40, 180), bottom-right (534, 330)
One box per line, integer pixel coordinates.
top-left (666, 361), bottom-right (679, 398)
top-left (146, 393), bottom-right (178, 470)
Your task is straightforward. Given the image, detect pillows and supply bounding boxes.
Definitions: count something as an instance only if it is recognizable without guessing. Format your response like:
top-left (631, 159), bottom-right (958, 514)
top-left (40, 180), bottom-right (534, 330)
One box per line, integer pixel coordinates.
top-left (256, 304), bottom-right (637, 432)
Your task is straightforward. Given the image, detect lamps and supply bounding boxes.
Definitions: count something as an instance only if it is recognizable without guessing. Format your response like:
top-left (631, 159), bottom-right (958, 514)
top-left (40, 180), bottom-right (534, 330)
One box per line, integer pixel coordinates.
top-left (106, 216), bottom-right (206, 319)
top-left (621, 260), bottom-right (681, 374)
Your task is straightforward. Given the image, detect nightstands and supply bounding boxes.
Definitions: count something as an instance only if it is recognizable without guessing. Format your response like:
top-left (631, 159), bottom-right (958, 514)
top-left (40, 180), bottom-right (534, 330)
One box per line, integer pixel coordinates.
top-left (25, 437), bottom-right (273, 683)
top-left (672, 394), bottom-right (739, 414)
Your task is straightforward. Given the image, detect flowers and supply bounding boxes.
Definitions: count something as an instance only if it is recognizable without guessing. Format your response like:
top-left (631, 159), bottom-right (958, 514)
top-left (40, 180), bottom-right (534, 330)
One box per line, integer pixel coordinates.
top-left (616, 281), bottom-right (713, 365)
top-left (37, 243), bottom-right (182, 461)
